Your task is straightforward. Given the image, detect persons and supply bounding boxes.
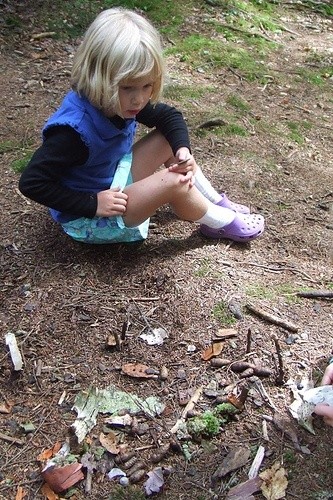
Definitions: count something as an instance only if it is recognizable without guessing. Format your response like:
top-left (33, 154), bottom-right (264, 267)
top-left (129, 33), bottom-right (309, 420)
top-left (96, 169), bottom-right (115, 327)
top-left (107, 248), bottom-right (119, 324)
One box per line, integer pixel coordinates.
top-left (19, 6), bottom-right (266, 247)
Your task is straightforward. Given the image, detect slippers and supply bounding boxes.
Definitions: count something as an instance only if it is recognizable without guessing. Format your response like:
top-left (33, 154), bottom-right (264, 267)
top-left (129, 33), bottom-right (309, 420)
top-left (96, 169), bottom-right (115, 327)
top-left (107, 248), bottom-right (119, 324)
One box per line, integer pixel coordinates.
top-left (200, 209), bottom-right (264, 242)
top-left (217, 193), bottom-right (250, 215)
